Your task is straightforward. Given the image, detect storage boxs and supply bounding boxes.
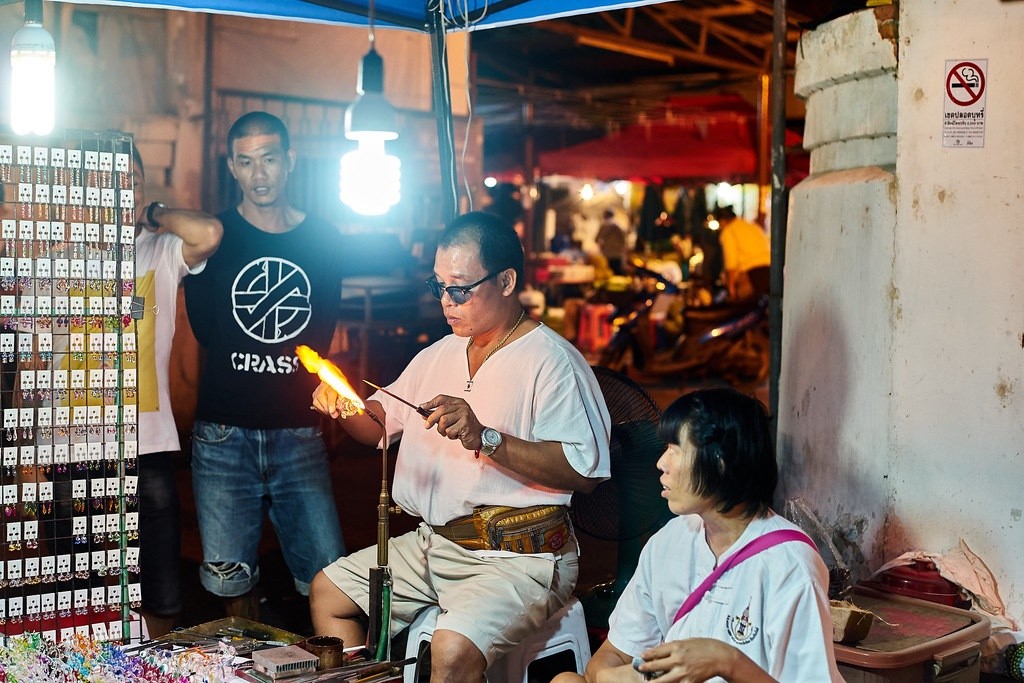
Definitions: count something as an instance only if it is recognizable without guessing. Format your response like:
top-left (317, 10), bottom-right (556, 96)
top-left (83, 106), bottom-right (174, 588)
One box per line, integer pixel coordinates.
top-left (834, 585), bottom-right (992, 683)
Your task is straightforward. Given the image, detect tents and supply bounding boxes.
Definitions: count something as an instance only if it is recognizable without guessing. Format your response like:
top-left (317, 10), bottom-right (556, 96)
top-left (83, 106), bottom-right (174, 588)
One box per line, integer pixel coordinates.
top-left (481, 111), bottom-right (811, 191)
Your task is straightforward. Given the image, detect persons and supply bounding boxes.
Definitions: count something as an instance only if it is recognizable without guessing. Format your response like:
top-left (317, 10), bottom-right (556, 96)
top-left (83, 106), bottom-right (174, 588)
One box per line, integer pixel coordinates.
top-left (182, 111), bottom-right (347, 621)
top-left (312, 210), bottom-right (612, 683)
top-left (549, 386), bottom-right (848, 683)
top-left (460, 182), bottom-right (772, 310)
top-left (0, 128), bottom-right (224, 642)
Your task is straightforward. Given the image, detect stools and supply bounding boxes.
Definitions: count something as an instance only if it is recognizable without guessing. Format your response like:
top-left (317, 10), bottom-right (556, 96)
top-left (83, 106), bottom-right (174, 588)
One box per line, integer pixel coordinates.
top-left (404, 600), bottom-right (592, 683)
top-left (577, 304), bottom-right (612, 353)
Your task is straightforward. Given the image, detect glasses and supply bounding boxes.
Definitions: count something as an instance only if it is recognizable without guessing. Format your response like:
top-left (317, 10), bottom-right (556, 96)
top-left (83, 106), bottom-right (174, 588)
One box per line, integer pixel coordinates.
top-left (425, 268), bottom-right (503, 304)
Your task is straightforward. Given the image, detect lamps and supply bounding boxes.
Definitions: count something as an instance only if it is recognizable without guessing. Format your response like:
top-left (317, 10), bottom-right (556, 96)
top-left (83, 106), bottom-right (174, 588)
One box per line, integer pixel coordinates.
top-left (9, 0), bottom-right (56, 136)
top-left (339, 0), bottom-right (401, 218)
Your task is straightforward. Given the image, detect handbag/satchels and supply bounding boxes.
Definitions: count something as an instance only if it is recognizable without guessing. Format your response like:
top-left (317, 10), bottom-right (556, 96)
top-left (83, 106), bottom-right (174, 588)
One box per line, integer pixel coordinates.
top-left (425, 503), bottom-right (572, 554)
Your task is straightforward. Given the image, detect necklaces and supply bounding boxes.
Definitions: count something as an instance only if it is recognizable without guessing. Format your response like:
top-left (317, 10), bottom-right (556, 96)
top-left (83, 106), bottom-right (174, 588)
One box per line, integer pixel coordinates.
top-left (464, 308), bottom-right (525, 391)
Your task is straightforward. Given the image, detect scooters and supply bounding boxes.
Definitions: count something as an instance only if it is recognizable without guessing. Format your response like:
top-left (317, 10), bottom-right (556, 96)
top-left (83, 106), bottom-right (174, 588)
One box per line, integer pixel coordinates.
top-left (598, 259), bottom-right (773, 390)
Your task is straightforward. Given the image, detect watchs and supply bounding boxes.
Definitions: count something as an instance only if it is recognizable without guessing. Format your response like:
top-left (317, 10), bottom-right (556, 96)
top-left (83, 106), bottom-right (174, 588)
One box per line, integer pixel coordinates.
top-left (475, 427), bottom-right (502, 460)
top-left (147, 200), bottom-right (165, 228)
